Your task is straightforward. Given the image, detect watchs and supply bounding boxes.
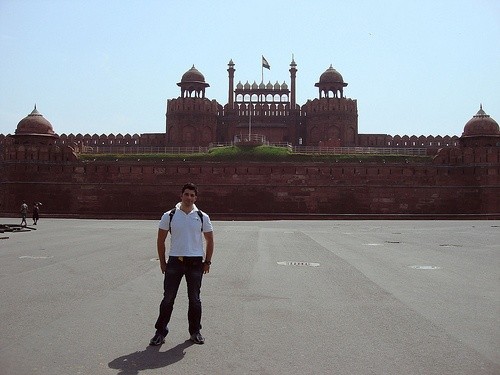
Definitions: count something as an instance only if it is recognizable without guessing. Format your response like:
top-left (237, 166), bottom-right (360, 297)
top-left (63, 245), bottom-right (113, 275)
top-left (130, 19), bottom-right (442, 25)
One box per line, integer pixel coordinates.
top-left (205, 260), bottom-right (211, 265)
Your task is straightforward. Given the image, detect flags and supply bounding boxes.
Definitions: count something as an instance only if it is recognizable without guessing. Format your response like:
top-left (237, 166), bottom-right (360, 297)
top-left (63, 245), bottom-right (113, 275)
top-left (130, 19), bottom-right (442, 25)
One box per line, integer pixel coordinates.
top-left (263, 56), bottom-right (270, 70)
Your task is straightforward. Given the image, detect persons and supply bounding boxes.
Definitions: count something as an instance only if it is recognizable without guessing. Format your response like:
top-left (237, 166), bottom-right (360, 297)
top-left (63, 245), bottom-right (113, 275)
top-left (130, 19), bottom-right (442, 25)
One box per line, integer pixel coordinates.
top-left (18, 200), bottom-right (28, 226)
top-left (32, 201), bottom-right (42, 225)
top-left (149, 182), bottom-right (214, 345)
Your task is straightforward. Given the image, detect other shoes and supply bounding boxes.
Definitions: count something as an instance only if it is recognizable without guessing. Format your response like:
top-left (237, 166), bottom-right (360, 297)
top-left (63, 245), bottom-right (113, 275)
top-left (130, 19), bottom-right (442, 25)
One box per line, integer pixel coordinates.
top-left (190, 332), bottom-right (205, 344)
top-left (150, 327), bottom-right (169, 346)
